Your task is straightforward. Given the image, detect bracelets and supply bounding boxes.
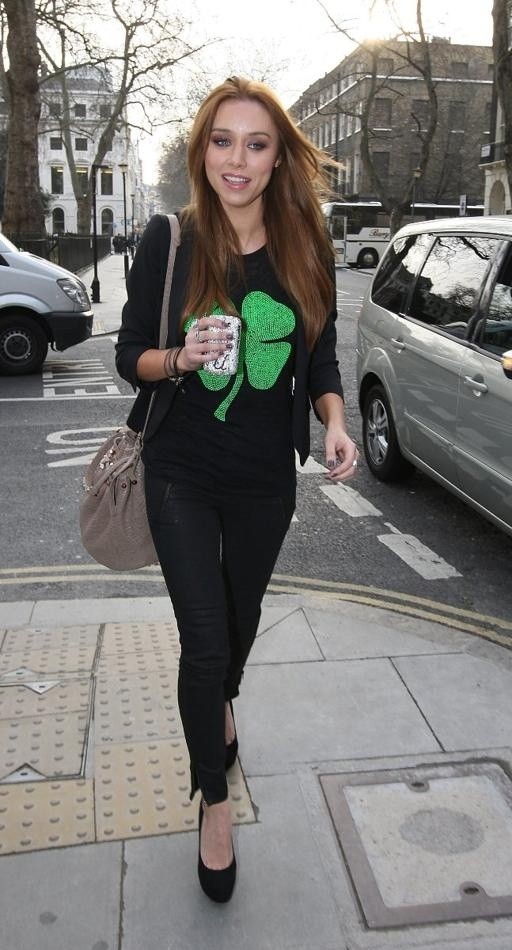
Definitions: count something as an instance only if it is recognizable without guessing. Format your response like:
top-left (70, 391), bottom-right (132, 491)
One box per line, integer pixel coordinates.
top-left (163, 345), bottom-right (186, 385)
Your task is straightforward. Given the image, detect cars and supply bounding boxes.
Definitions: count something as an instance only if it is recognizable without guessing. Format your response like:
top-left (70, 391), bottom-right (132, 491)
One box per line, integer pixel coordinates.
top-left (356, 216), bottom-right (511, 535)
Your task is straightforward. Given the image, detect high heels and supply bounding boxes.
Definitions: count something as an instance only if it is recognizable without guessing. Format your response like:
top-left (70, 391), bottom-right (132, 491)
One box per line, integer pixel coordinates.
top-left (224, 695), bottom-right (238, 772)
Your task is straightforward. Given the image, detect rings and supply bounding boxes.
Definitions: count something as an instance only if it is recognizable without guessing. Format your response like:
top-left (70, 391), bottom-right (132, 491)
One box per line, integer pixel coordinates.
top-left (193, 319), bottom-right (200, 330)
top-left (353, 459), bottom-right (357, 467)
top-left (194, 330), bottom-right (202, 343)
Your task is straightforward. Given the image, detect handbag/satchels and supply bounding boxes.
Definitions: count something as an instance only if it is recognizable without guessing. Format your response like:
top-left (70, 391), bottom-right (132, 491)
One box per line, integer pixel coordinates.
top-left (78, 430), bottom-right (160, 571)
top-left (197, 797), bottom-right (237, 904)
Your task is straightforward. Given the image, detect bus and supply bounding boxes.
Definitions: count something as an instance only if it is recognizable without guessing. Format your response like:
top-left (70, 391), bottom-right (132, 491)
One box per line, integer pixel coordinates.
top-left (320, 201), bottom-right (483, 268)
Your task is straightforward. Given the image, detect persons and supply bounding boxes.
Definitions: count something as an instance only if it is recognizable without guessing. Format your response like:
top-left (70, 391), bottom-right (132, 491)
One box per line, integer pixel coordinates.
top-left (115, 73), bottom-right (362, 903)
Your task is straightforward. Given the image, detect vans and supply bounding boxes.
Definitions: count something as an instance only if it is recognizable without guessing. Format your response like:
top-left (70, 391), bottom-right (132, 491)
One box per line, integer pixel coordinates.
top-left (1, 234), bottom-right (92, 377)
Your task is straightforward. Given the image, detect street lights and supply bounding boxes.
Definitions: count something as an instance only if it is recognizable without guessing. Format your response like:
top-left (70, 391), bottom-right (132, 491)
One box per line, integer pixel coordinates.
top-left (90, 162), bottom-right (130, 302)
